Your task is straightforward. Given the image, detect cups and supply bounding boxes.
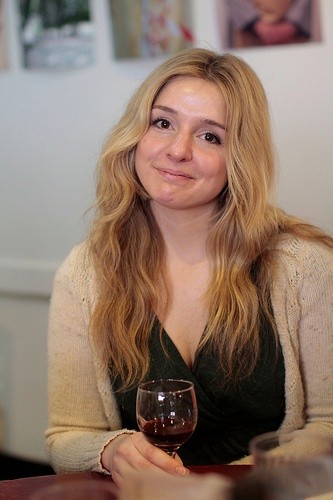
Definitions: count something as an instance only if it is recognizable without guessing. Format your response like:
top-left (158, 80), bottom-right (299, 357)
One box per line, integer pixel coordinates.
top-left (136, 380), bottom-right (198, 458)
top-left (250, 432), bottom-right (281, 467)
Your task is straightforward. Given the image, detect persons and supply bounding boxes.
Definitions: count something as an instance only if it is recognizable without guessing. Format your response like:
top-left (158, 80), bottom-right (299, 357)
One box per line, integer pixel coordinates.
top-left (221, 0), bottom-right (321, 48)
top-left (42, 47), bottom-right (332, 499)
